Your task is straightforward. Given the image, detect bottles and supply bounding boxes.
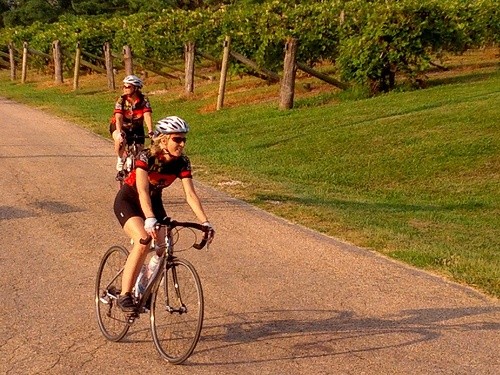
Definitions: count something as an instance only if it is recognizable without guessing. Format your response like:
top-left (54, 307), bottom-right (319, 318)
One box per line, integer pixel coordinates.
top-left (127, 155), bottom-right (131, 171)
top-left (138, 255), bottom-right (160, 292)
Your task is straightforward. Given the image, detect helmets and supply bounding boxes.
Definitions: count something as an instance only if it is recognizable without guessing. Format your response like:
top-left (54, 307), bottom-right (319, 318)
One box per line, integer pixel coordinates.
top-left (155, 115), bottom-right (189, 133)
top-left (123, 75), bottom-right (143, 88)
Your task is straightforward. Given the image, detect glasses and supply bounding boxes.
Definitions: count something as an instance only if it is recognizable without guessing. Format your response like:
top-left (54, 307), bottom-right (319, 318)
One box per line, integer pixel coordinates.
top-left (123, 85), bottom-right (134, 89)
top-left (166, 137), bottom-right (187, 143)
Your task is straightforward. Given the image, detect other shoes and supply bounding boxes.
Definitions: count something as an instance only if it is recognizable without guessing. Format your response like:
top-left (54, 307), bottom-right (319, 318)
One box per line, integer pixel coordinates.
top-left (116, 157), bottom-right (124, 171)
top-left (134, 288), bottom-right (153, 311)
top-left (125, 157), bottom-right (132, 171)
top-left (117, 292), bottom-right (136, 313)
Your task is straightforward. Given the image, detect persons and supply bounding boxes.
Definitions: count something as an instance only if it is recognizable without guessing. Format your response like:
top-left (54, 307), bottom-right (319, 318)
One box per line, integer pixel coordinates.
top-left (110, 75), bottom-right (155, 171)
top-left (113, 116), bottom-right (214, 312)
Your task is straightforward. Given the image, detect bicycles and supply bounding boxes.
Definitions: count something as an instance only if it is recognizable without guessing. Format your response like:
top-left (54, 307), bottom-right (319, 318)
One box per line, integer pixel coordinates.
top-left (117, 129), bottom-right (156, 188)
top-left (94, 216), bottom-right (215, 364)
